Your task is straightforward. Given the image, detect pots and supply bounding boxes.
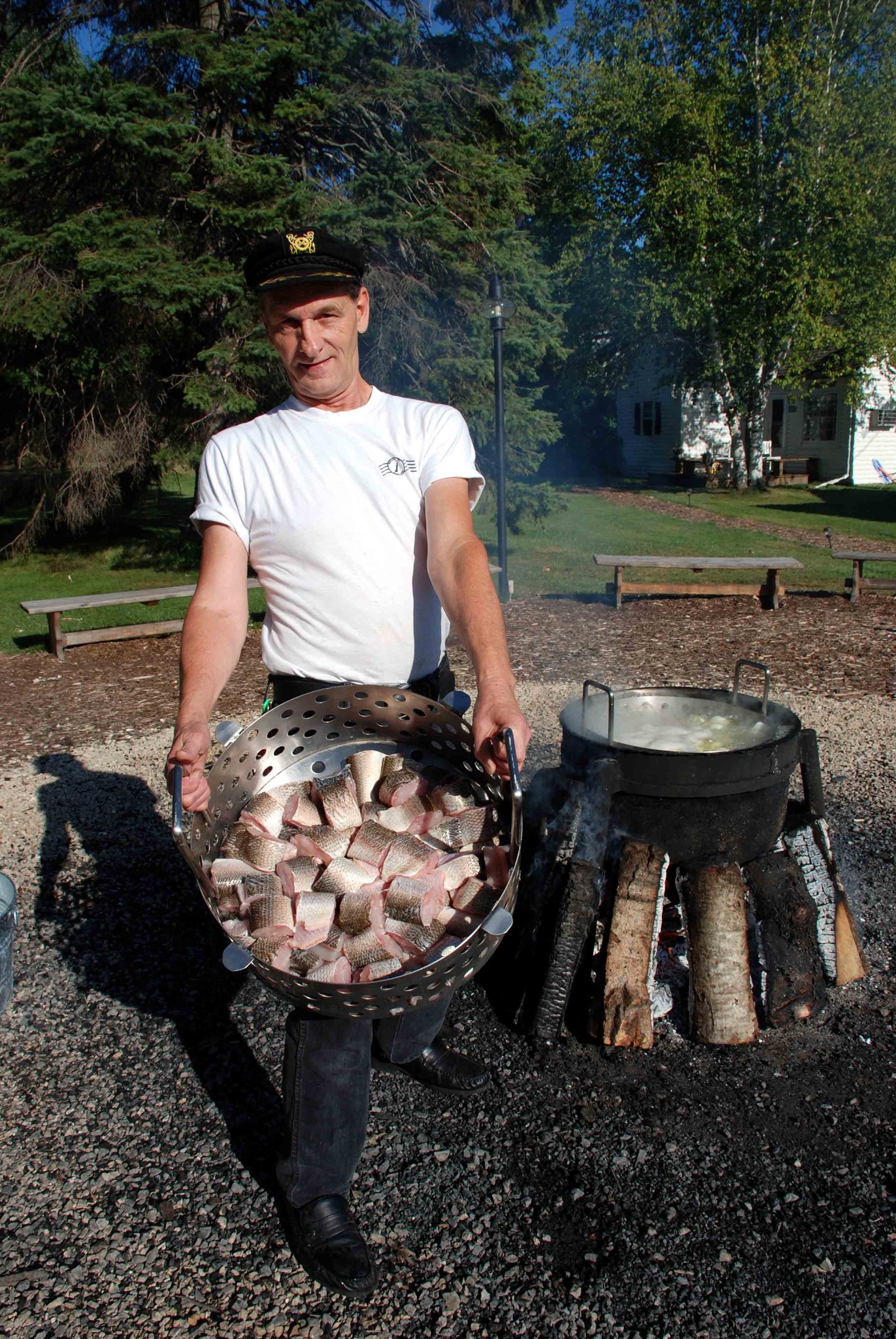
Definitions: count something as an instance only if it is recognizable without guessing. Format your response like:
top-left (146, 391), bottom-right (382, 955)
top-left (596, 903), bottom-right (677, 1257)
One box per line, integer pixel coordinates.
top-left (555, 659), bottom-right (801, 872)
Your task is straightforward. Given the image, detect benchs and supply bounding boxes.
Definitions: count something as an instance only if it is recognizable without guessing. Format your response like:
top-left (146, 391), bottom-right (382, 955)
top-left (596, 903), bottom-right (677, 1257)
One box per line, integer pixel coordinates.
top-left (24, 577), bottom-right (264, 663)
top-left (592, 554), bottom-right (803, 610)
top-left (831, 551), bottom-right (896, 602)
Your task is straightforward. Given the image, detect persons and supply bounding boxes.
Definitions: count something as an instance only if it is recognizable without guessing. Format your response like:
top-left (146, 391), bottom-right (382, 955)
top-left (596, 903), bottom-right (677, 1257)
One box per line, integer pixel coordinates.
top-left (164, 226), bottom-right (532, 1298)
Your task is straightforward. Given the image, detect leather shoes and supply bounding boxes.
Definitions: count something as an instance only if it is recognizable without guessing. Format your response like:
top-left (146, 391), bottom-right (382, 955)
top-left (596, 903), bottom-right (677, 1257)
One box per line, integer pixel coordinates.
top-left (376, 1037), bottom-right (491, 1096)
top-left (289, 1187), bottom-right (375, 1298)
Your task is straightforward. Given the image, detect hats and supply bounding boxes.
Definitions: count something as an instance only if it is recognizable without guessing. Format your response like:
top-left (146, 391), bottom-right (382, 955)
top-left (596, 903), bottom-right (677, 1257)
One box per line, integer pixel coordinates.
top-left (243, 225), bottom-right (364, 294)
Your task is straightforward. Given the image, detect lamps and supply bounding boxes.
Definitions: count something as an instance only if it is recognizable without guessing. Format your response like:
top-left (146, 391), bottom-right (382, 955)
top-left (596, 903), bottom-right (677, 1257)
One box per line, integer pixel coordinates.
top-left (789, 404), bottom-right (798, 413)
top-left (711, 403), bottom-right (718, 415)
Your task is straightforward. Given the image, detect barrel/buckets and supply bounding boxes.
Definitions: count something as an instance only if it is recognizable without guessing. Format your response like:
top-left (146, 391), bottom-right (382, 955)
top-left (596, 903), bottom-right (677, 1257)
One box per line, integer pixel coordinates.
top-left (0, 871), bottom-right (18, 1015)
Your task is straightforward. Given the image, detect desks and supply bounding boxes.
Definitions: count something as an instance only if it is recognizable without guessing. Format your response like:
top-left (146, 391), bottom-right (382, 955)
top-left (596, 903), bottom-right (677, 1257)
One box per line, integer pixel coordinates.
top-left (681, 457), bottom-right (733, 487)
top-left (766, 457), bottom-right (810, 477)
